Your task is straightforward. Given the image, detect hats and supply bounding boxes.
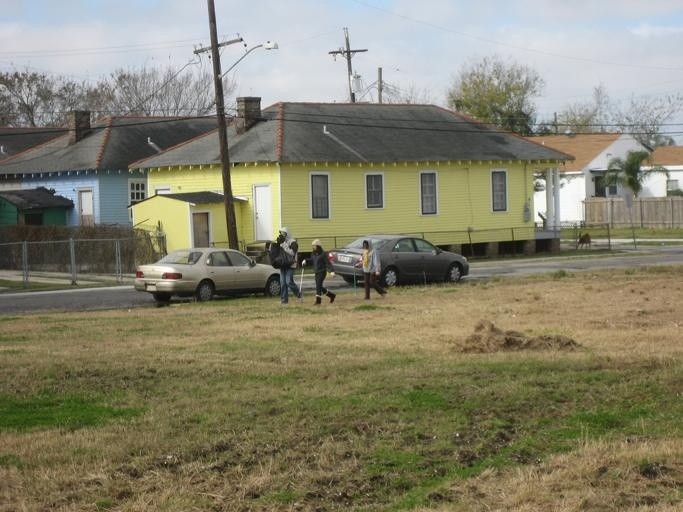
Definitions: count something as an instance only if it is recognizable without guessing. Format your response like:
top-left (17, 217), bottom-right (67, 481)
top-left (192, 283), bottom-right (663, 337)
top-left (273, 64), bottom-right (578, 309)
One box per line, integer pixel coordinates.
top-left (312, 239), bottom-right (321, 245)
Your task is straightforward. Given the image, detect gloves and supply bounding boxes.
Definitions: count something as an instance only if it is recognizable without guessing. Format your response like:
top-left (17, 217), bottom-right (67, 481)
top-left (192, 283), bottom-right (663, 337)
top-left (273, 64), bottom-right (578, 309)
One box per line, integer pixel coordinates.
top-left (302, 259), bottom-right (307, 266)
top-left (330, 272), bottom-right (336, 279)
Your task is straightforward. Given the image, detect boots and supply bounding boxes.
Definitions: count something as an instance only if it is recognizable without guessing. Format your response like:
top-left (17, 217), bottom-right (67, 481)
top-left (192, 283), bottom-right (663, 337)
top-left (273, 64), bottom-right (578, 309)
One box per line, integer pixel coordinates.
top-left (313, 295), bottom-right (322, 306)
top-left (324, 289), bottom-right (336, 303)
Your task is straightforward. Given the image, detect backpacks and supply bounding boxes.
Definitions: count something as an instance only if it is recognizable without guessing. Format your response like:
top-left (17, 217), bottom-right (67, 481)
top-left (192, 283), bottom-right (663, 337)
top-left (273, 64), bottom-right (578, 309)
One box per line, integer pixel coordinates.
top-left (268, 242), bottom-right (295, 269)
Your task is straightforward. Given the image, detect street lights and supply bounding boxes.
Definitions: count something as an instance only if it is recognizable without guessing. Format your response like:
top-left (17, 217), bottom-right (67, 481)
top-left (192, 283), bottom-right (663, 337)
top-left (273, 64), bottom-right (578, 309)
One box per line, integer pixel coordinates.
top-left (212, 41), bottom-right (279, 249)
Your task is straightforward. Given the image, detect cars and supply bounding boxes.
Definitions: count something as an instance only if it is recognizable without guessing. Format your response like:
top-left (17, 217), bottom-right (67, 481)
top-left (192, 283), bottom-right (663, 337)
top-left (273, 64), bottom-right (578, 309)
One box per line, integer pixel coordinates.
top-left (327, 233), bottom-right (469, 288)
top-left (134, 248), bottom-right (288, 303)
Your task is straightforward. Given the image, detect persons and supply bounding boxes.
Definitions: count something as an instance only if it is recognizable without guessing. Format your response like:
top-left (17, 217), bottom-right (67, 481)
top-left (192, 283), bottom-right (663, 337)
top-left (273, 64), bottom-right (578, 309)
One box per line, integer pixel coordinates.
top-left (274, 227), bottom-right (302, 304)
top-left (301, 238), bottom-right (336, 306)
top-left (360, 239), bottom-right (387, 300)
